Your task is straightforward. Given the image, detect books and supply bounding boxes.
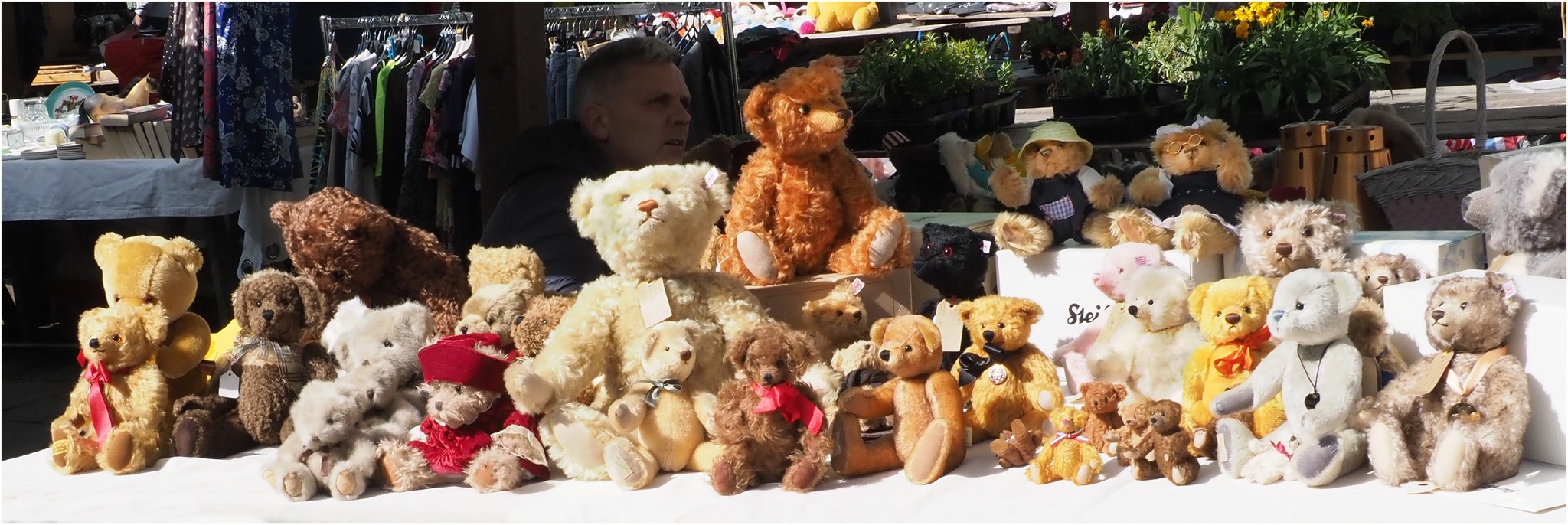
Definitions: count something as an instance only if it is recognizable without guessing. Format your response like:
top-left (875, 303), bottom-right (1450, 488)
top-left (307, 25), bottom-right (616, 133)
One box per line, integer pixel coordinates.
top-left (68, 104), bottom-right (203, 160)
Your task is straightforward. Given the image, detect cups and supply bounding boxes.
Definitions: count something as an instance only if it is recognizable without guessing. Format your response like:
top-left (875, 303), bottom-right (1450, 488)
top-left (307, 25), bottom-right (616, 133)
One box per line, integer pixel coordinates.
top-left (16, 97), bottom-right (46, 122)
top-left (45, 129), bottom-right (67, 146)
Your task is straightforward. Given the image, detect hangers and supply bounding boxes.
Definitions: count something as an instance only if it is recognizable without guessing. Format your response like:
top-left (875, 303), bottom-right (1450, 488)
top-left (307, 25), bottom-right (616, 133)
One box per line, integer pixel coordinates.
top-left (327, 2), bottom-right (708, 67)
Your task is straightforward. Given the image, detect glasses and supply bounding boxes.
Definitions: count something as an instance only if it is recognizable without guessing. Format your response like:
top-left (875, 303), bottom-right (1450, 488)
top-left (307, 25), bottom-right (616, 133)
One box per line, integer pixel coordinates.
top-left (1165, 134), bottom-right (1204, 154)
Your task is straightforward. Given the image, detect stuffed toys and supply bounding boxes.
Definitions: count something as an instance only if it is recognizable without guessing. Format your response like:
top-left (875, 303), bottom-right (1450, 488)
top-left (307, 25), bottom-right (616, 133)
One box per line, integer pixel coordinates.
top-left (701, 172), bottom-right (717, 174)
top-left (206, 266), bottom-right (338, 445)
top-left (50, 302), bottom-right (170, 476)
top-left (701, 54), bottom-right (912, 286)
top-left (95, 232), bottom-right (210, 458)
top-left (676, 2), bottom-right (879, 42)
top-left (263, 115), bottom-right (1432, 502)
top-left (1349, 270), bottom-right (1531, 491)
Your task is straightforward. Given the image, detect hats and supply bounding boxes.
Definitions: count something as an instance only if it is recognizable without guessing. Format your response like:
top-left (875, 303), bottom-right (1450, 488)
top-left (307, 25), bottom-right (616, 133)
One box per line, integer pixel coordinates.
top-left (1017, 122), bottom-right (1093, 163)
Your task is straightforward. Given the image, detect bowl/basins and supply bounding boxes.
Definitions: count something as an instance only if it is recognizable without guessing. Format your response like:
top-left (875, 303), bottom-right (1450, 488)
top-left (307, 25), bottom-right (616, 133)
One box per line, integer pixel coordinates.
top-left (20, 121), bottom-right (59, 138)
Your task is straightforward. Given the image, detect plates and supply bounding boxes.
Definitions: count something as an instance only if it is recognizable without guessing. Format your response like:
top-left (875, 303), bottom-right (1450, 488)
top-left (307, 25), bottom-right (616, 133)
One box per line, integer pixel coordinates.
top-left (46, 81), bottom-right (96, 119)
top-left (21, 142), bottom-right (86, 160)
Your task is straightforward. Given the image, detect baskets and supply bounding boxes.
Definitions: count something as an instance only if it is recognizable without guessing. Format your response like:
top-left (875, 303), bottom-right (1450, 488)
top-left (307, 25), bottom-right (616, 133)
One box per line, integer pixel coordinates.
top-left (1356, 28), bottom-right (1509, 230)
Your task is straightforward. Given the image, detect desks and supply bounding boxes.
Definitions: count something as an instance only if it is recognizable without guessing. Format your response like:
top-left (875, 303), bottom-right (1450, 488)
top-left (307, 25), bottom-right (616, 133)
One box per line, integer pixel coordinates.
top-left (31, 63), bottom-right (119, 97)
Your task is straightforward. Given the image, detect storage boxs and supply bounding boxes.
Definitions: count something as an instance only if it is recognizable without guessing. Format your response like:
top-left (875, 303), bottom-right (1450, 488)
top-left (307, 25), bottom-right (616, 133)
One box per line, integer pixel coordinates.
top-left (747, 210), bottom-right (1568, 469)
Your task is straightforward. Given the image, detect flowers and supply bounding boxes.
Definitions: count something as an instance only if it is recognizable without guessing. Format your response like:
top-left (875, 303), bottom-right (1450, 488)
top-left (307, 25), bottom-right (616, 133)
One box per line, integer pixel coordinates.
top-left (1040, 20), bottom-right (1158, 101)
top-left (1174, 1), bottom-right (1394, 124)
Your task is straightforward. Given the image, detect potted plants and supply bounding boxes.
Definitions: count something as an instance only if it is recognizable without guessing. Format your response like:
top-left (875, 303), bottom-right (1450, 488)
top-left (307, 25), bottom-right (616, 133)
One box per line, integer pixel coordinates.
top-left (842, 29), bottom-right (1021, 145)
top-left (1137, 2), bottom-right (1220, 103)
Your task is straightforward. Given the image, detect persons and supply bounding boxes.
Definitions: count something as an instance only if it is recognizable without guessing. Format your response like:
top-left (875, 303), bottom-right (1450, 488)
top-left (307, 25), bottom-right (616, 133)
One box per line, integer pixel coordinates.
top-left (480, 36), bottom-right (691, 297)
top-left (139, 2), bottom-right (173, 37)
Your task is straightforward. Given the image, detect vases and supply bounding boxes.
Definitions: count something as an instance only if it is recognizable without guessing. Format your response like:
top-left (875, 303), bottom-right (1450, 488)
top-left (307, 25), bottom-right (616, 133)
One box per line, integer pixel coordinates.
top-left (1048, 89), bottom-right (1145, 118)
top-left (1218, 81), bottom-right (1371, 141)
